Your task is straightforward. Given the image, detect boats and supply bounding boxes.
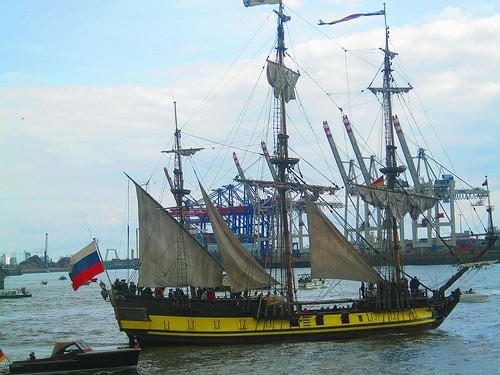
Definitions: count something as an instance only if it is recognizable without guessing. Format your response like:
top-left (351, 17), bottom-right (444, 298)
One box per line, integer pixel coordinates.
top-left (40, 281), bottom-right (48, 285)
top-left (459, 288), bottom-right (490, 303)
top-left (297, 273), bottom-right (326, 290)
top-left (0, 338), bottom-right (142, 375)
top-left (71, 282), bottom-right (91, 285)
top-left (91, 278), bottom-right (98, 282)
top-left (0, 287), bottom-right (32, 298)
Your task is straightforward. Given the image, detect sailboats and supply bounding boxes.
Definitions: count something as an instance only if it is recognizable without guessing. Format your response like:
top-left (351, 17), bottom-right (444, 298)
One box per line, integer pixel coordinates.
top-left (69, 0), bottom-right (462, 347)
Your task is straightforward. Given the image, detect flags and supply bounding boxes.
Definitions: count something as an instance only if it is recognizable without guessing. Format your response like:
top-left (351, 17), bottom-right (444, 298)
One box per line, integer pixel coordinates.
top-left (69, 241), bottom-right (104, 292)
top-left (0, 349), bottom-right (8, 368)
top-left (370, 175), bottom-right (384, 186)
top-left (438, 213), bottom-right (444, 218)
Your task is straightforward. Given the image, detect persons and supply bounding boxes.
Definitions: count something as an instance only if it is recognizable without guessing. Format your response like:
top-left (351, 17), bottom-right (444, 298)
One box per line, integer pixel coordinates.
top-left (360, 276), bottom-right (419, 299)
top-left (113, 278), bottom-right (284, 302)
top-left (468, 288), bottom-right (472, 292)
top-left (29, 352), bottom-right (36, 360)
top-left (298, 276), bottom-right (312, 283)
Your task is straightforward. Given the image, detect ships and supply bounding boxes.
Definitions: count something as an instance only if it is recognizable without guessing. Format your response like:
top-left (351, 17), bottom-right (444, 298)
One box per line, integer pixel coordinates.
top-left (101, 100), bottom-right (500, 269)
